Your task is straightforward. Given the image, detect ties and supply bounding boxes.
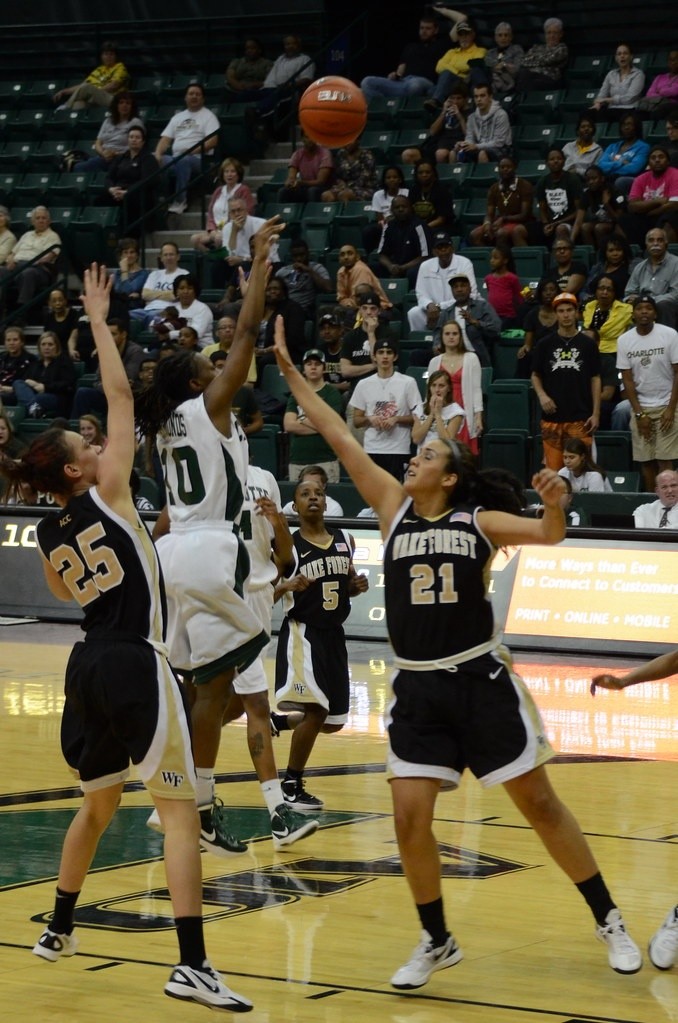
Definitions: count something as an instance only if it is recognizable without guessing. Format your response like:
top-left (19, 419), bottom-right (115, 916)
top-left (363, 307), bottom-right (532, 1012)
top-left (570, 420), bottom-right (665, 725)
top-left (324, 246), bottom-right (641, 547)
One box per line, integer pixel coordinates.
top-left (659, 507), bottom-right (671, 527)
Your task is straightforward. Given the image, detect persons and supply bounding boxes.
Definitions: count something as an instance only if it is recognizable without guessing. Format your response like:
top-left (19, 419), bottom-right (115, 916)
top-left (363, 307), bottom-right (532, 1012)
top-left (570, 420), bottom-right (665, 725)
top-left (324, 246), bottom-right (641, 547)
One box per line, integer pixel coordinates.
top-left (273, 313), bottom-right (641, 991)
top-left (0, 261), bottom-right (252, 1012)
top-left (590, 648), bottom-right (678, 970)
top-left (0, 0), bottom-right (678, 854)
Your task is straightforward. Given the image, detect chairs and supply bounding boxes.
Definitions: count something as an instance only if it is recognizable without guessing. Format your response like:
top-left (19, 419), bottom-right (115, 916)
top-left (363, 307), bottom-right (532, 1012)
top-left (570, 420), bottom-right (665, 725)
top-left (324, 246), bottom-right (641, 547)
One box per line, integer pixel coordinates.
top-left (0, 0), bottom-right (678, 536)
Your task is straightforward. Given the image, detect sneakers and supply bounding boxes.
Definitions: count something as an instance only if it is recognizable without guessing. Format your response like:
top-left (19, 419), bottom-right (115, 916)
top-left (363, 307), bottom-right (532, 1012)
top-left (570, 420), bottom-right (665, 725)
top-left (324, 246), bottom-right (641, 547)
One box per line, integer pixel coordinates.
top-left (391, 928), bottom-right (463, 989)
top-left (595, 909), bottom-right (642, 974)
top-left (146, 807), bottom-right (165, 834)
top-left (271, 803), bottom-right (319, 851)
top-left (32, 926), bottom-right (78, 962)
top-left (280, 780), bottom-right (324, 809)
top-left (164, 959), bottom-right (254, 1014)
top-left (270, 710), bottom-right (280, 737)
top-left (648, 904), bottom-right (678, 970)
top-left (197, 796), bottom-right (248, 858)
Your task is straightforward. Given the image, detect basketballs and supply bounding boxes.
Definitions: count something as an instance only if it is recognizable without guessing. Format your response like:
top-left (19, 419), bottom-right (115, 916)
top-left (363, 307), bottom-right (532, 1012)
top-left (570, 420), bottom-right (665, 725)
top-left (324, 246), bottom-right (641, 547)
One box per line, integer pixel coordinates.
top-left (299, 76), bottom-right (367, 149)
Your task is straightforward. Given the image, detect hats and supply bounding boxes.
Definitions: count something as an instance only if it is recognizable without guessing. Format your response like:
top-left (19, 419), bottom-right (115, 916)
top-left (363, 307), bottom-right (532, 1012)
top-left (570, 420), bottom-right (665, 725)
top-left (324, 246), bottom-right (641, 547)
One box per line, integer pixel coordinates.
top-left (431, 232), bottom-right (452, 247)
top-left (358, 293), bottom-right (380, 306)
top-left (303, 349), bottom-right (325, 366)
top-left (374, 339), bottom-right (396, 354)
top-left (317, 313), bottom-right (340, 326)
top-left (633, 295), bottom-right (656, 308)
top-left (552, 292), bottom-right (578, 309)
top-left (448, 273), bottom-right (470, 285)
top-left (456, 20), bottom-right (477, 32)
